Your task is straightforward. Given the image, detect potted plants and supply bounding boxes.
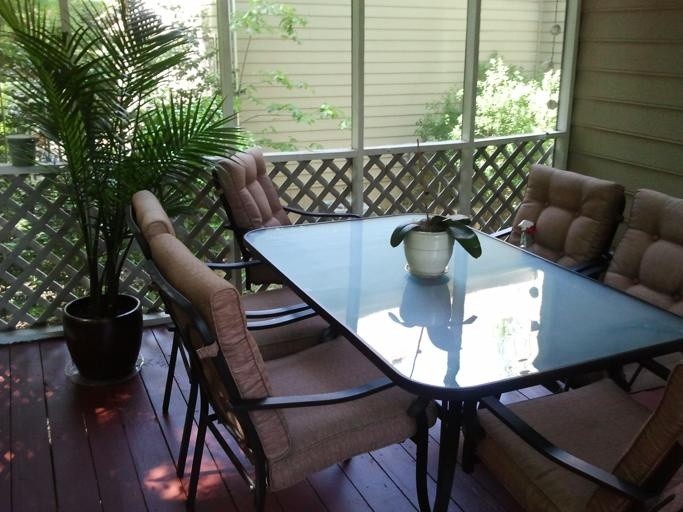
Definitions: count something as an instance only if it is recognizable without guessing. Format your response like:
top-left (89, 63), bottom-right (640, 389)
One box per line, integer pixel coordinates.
top-left (2, 2), bottom-right (237, 388)
top-left (389, 205), bottom-right (480, 280)
